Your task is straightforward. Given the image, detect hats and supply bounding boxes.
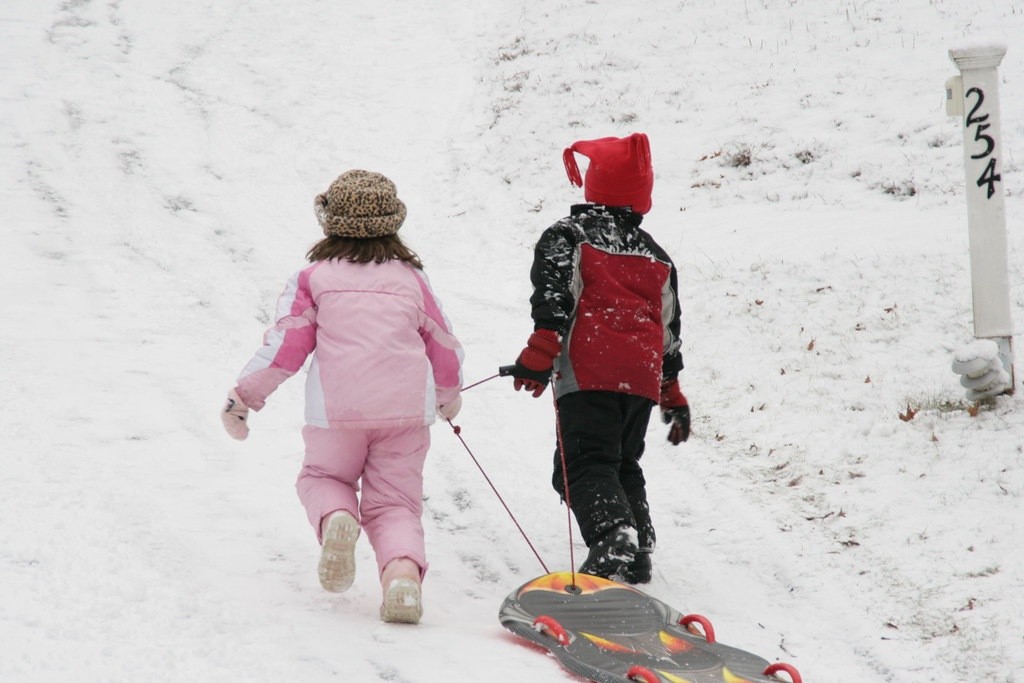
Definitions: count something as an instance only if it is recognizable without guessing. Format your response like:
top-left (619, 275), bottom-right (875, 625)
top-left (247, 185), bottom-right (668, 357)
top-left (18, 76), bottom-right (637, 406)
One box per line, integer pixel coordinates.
top-left (564, 133), bottom-right (653, 213)
top-left (314, 169), bottom-right (406, 237)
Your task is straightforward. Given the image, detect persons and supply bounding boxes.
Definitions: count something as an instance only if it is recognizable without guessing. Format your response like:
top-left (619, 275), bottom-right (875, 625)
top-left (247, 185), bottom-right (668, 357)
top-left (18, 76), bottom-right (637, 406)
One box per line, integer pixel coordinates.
top-left (221, 170), bottom-right (463, 624)
top-left (515, 134), bottom-right (691, 583)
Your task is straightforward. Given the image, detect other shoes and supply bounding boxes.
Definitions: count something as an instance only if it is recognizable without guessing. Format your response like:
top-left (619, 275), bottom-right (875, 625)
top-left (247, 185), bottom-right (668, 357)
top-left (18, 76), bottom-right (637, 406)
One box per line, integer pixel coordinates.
top-left (319, 509), bottom-right (360, 594)
top-left (578, 524), bottom-right (639, 581)
top-left (620, 551), bottom-right (652, 584)
top-left (380, 557), bottom-right (422, 624)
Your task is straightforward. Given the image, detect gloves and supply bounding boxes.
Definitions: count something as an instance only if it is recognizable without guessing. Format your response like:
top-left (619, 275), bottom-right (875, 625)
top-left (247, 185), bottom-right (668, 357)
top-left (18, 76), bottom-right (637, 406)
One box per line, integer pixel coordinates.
top-left (437, 393), bottom-right (462, 421)
top-left (513, 328), bottom-right (563, 398)
top-left (658, 379), bottom-right (690, 445)
top-left (221, 386), bottom-right (251, 440)
